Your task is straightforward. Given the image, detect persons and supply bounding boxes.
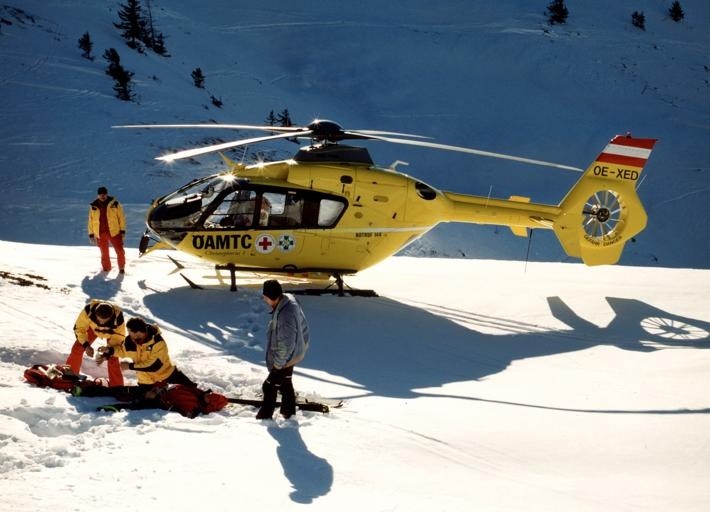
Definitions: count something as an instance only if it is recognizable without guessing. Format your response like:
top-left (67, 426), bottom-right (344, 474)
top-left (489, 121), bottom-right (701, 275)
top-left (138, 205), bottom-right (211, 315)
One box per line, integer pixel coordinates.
top-left (69, 383), bottom-right (229, 419)
top-left (87, 187), bottom-right (127, 274)
top-left (66, 299), bottom-right (126, 387)
top-left (97, 319), bottom-right (198, 410)
top-left (255, 280), bottom-right (311, 418)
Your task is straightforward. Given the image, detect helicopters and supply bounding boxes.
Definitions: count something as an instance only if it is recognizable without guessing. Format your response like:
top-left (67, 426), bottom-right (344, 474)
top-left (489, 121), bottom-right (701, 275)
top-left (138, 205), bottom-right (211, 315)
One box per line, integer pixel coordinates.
top-left (110, 119), bottom-right (658, 297)
